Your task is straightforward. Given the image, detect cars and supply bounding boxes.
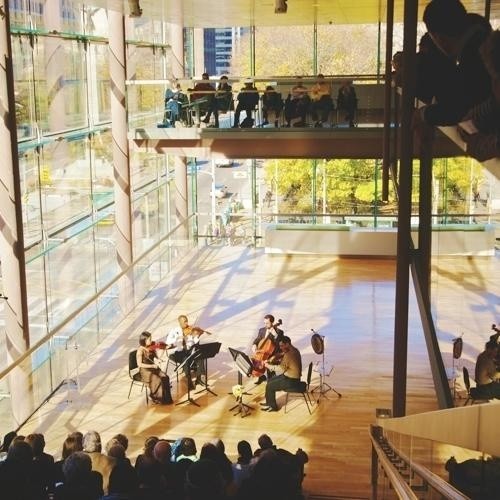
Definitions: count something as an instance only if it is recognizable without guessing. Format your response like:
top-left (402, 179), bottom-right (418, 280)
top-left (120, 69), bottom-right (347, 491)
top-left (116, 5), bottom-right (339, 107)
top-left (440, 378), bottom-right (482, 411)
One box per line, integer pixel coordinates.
top-left (216, 157), bottom-right (235, 168)
top-left (210, 184), bottom-right (230, 199)
top-left (96, 213), bottom-right (114, 226)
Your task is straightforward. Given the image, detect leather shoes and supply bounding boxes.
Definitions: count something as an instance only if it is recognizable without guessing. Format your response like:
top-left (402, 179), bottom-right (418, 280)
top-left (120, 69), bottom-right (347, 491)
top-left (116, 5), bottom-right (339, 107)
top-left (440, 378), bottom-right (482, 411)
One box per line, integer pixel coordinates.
top-left (261, 406), bottom-right (278, 412)
top-left (260, 403), bottom-right (269, 406)
top-left (195, 379), bottom-right (209, 386)
top-left (254, 375), bottom-right (266, 384)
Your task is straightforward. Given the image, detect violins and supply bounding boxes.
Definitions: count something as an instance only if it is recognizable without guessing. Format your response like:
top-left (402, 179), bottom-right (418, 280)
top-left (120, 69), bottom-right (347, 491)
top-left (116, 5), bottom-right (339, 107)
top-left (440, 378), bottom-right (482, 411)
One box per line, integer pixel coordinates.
top-left (146, 341), bottom-right (176, 351)
top-left (258, 354), bottom-right (285, 369)
top-left (183, 326), bottom-right (210, 336)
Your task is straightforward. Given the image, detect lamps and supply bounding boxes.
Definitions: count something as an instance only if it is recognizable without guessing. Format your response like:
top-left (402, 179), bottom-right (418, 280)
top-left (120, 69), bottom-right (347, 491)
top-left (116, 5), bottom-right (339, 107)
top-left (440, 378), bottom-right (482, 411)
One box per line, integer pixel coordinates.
top-left (273, 0), bottom-right (290, 14)
top-left (128, 1), bottom-right (143, 19)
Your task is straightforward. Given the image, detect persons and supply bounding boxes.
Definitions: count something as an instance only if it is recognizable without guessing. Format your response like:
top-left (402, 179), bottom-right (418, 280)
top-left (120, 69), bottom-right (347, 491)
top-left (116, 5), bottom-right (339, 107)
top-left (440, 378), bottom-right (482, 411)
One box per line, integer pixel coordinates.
top-left (251, 314), bottom-right (284, 387)
top-left (258, 336), bottom-right (301, 413)
top-left (445, 452), bottom-right (500, 500)
top-left (135, 331), bottom-right (175, 405)
top-left (164, 314), bottom-right (209, 391)
top-left (155, 69), bottom-right (359, 127)
top-left (0, 430), bottom-right (311, 500)
top-left (474, 341), bottom-right (500, 401)
top-left (386, 1), bottom-right (500, 172)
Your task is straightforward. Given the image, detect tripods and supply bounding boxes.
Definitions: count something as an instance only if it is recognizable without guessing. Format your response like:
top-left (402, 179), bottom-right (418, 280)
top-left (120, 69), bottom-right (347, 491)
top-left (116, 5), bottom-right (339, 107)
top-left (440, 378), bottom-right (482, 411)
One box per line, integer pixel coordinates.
top-left (195, 357), bottom-right (218, 396)
top-left (228, 370), bottom-right (255, 418)
top-left (311, 337), bottom-right (341, 403)
top-left (176, 331), bottom-right (204, 407)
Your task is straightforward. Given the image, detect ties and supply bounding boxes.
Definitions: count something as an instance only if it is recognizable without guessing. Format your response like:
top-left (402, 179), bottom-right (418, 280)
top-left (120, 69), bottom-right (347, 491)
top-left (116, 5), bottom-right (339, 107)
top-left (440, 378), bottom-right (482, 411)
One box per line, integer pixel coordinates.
top-left (182, 331), bottom-right (187, 351)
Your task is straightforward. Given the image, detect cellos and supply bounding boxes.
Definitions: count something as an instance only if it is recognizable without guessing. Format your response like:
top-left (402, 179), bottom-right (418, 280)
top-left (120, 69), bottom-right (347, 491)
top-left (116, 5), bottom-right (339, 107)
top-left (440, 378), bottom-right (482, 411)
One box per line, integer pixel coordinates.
top-left (251, 319), bottom-right (282, 377)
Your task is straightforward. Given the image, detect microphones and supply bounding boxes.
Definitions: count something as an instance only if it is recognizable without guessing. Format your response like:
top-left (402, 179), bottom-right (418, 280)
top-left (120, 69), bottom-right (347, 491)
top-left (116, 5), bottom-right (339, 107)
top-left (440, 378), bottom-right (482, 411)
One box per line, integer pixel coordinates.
top-left (205, 331), bottom-right (211, 335)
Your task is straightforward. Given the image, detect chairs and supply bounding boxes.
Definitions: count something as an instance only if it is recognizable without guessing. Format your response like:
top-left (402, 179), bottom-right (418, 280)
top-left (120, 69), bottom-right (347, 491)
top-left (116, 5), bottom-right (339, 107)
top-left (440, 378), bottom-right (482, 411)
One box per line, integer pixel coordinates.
top-left (166, 85), bottom-right (362, 128)
top-left (459, 366), bottom-right (493, 406)
top-left (126, 336), bottom-right (317, 415)
